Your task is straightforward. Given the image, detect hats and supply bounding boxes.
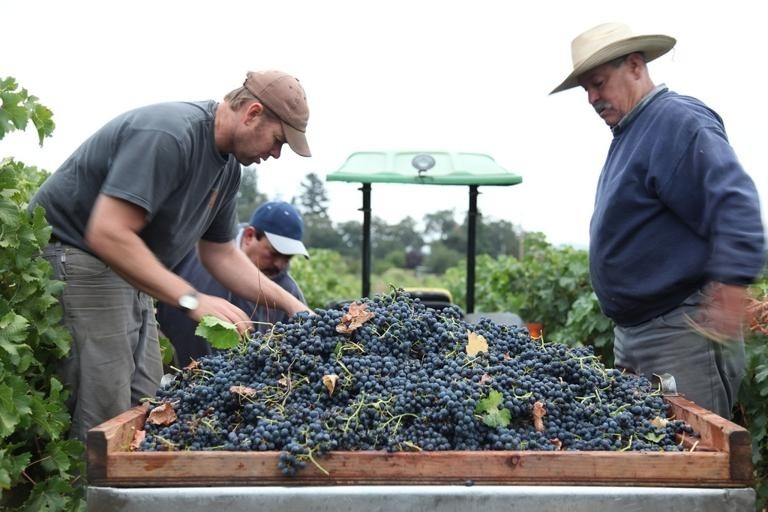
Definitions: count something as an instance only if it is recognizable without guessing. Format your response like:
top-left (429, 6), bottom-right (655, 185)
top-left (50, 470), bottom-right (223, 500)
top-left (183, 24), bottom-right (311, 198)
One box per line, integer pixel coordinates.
top-left (248, 201), bottom-right (309, 260)
top-left (243, 70), bottom-right (311, 157)
top-left (548, 20), bottom-right (677, 96)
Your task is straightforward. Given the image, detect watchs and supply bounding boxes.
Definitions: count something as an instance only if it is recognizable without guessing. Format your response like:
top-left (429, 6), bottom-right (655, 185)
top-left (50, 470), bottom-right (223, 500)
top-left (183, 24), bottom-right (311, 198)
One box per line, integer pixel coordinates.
top-left (175, 288), bottom-right (201, 313)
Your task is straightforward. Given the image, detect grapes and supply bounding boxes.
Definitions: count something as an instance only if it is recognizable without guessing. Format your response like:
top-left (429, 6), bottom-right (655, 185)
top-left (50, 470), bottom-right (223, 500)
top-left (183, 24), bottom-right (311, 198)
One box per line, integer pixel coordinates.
top-left (135, 283), bottom-right (702, 477)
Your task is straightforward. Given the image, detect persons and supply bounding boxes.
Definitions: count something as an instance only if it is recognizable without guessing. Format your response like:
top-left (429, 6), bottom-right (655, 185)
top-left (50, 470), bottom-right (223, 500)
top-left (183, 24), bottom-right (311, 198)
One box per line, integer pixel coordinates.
top-left (578, 51), bottom-right (768, 421)
top-left (156, 201), bottom-right (308, 375)
top-left (22, 72), bottom-right (316, 497)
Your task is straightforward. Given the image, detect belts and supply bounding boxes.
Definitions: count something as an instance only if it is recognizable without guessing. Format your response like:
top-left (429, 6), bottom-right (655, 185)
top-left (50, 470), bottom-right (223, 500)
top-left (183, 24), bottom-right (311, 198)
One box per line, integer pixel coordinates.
top-left (49, 232), bottom-right (57, 243)
